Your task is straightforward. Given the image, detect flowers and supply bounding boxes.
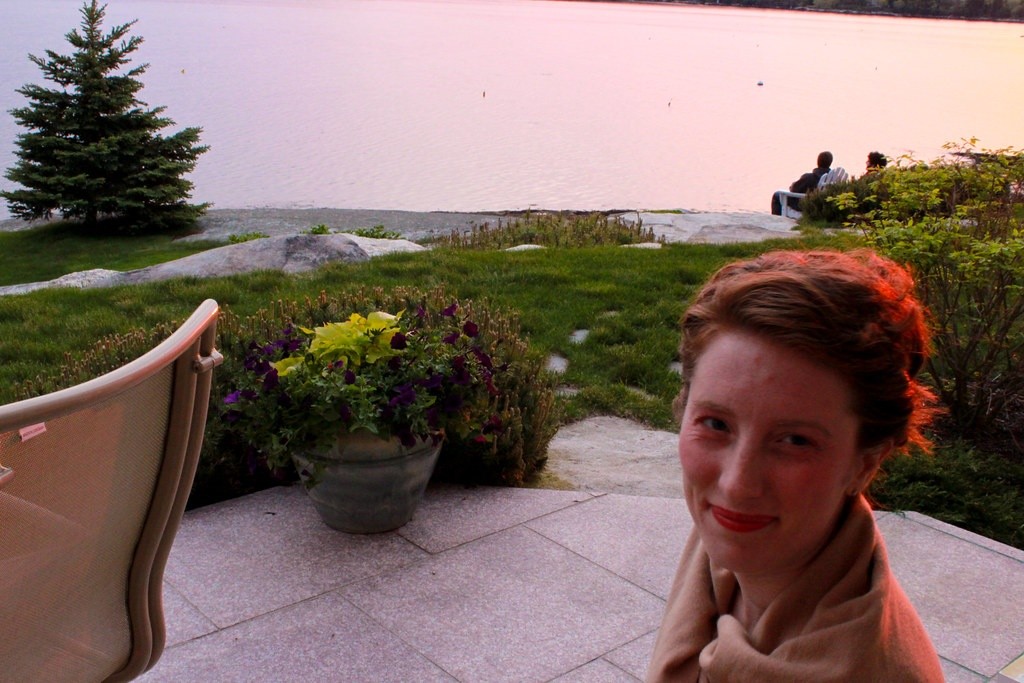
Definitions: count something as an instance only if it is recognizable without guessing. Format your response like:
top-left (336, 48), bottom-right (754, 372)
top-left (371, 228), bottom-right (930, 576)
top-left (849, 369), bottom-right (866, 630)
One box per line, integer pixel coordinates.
top-left (221, 304), bottom-right (509, 490)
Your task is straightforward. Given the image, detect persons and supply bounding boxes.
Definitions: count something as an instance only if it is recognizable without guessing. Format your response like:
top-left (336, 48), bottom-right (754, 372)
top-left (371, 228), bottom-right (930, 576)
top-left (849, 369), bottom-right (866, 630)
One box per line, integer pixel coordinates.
top-left (787, 151), bottom-right (832, 212)
top-left (860, 151), bottom-right (886, 178)
top-left (646, 247), bottom-right (947, 683)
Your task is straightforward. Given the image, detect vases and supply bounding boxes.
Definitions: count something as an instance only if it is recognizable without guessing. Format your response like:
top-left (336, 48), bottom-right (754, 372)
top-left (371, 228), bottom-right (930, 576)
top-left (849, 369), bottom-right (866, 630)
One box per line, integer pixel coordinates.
top-left (291, 433), bottom-right (444, 538)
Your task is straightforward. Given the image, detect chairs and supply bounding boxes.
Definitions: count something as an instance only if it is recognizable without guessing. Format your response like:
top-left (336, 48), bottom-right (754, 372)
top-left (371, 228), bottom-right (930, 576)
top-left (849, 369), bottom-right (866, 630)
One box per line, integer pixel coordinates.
top-left (0, 297), bottom-right (225, 682)
top-left (777, 168), bottom-right (851, 221)
